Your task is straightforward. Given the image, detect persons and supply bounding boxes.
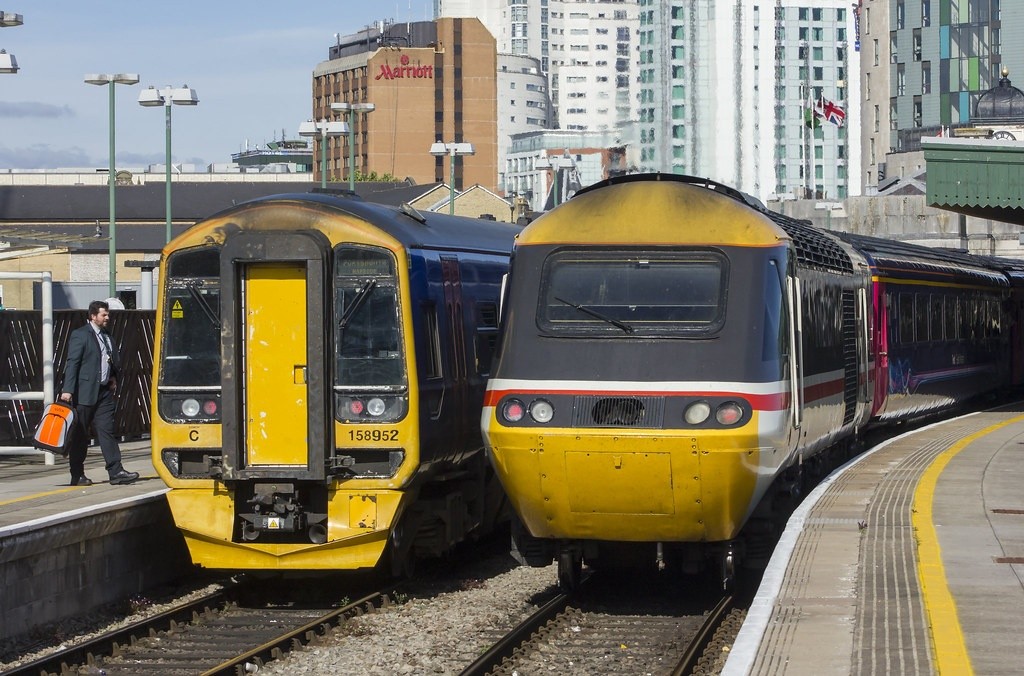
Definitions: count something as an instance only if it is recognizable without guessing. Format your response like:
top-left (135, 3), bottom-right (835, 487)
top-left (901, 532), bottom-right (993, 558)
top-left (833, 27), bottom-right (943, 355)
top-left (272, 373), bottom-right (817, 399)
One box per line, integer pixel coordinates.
top-left (58, 300), bottom-right (139, 486)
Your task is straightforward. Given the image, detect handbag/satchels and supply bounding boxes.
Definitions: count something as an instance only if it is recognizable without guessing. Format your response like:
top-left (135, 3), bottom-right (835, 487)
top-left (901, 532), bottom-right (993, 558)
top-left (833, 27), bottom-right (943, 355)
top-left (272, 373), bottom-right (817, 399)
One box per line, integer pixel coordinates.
top-left (32, 397), bottom-right (78, 458)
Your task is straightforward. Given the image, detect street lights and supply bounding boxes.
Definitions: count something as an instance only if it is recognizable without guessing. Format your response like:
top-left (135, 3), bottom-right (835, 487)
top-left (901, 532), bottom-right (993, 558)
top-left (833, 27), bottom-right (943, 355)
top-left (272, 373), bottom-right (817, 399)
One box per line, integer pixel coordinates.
top-left (428, 140), bottom-right (477, 216)
top-left (297, 118), bottom-right (351, 188)
top-left (136, 83), bottom-right (200, 247)
top-left (83, 73), bottom-right (141, 298)
top-left (534, 155), bottom-right (577, 207)
top-left (813, 200), bottom-right (843, 231)
top-left (330, 102), bottom-right (376, 192)
top-left (766, 192), bottom-right (798, 215)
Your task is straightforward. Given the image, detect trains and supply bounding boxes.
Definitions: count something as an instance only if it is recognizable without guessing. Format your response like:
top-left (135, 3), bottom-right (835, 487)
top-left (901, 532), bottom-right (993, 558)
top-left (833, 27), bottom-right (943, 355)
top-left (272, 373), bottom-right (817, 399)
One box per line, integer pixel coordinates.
top-left (147, 192), bottom-right (529, 573)
top-left (477, 171), bottom-right (1024, 592)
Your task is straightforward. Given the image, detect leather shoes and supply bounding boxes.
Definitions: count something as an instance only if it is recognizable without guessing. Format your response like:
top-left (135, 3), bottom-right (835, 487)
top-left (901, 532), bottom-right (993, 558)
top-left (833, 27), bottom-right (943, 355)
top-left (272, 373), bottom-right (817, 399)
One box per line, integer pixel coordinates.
top-left (71, 473), bottom-right (92, 486)
top-left (110, 470), bottom-right (139, 485)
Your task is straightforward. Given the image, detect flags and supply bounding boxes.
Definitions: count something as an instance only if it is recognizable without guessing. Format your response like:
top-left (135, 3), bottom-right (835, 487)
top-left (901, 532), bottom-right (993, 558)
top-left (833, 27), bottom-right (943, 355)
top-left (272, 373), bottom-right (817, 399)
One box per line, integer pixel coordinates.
top-left (805, 91), bottom-right (845, 130)
